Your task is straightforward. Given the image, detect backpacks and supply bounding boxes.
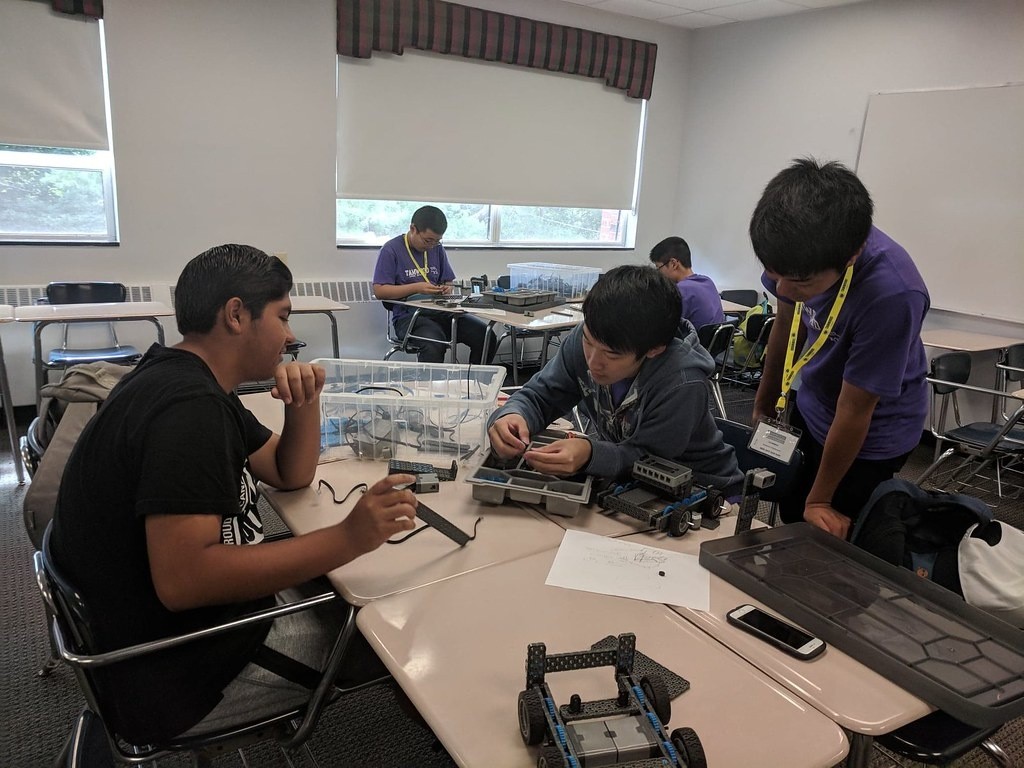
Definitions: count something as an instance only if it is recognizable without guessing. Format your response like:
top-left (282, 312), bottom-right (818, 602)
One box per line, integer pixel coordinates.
top-left (23, 362), bottom-right (136, 551)
top-left (849, 479), bottom-right (991, 604)
top-left (733, 292), bottom-right (773, 369)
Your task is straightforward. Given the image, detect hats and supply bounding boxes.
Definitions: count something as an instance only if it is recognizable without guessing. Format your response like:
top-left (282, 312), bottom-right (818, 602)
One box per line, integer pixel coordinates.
top-left (957, 520), bottom-right (1024, 630)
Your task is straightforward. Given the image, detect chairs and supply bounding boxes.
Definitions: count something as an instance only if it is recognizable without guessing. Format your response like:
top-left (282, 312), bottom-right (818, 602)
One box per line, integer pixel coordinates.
top-left (995, 344), bottom-right (1024, 477)
top-left (380, 302), bottom-right (451, 362)
top-left (719, 289), bottom-right (758, 323)
top-left (715, 312), bottom-right (775, 420)
top-left (18, 282), bottom-right (362, 757)
top-left (714, 416), bottom-right (784, 526)
top-left (698, 318), bottom-right (740, 420)
top-left (926, 350), bottom-right (1024, 507)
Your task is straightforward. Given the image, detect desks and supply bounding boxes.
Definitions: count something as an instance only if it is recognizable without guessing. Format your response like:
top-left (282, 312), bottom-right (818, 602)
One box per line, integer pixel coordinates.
top-left (239, 292), bottom-right (1024, 768)
top-left (0, 304), bottom-right (27, 485)
top-left (15, 302), bottom-right (175, 416)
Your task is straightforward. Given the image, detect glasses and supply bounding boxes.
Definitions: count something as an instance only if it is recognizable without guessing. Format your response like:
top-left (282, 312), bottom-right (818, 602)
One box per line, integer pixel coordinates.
top-left (413, 225), bottom-right (443, 247)
top-left (654, 258), bottom-right (678, 270)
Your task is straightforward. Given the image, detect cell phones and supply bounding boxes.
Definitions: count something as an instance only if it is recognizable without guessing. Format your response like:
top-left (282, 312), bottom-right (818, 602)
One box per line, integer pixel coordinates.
top-left (726, 604), bottom-right (826, 660)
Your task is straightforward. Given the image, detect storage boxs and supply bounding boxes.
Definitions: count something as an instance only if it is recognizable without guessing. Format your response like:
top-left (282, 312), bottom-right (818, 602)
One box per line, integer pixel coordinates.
top-left (308, 358), bottom-right (507, 467)
top-left (481, 262), bottom-right (602, 306)
top-left (465, 429), bottom-right (596, 519)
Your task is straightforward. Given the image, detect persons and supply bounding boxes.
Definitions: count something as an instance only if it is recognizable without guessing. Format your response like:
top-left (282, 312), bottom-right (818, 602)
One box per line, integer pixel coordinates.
top-left (751, 157), bottom-right (930, 540)
top-left (50, 245), bottom-right (418, 740)
top-left (649, 237), bottom-right (724, 333)
top-left (487, 266), bottom-right (744, 499)
top-left (372, 205), bottom-right (497, 365)
